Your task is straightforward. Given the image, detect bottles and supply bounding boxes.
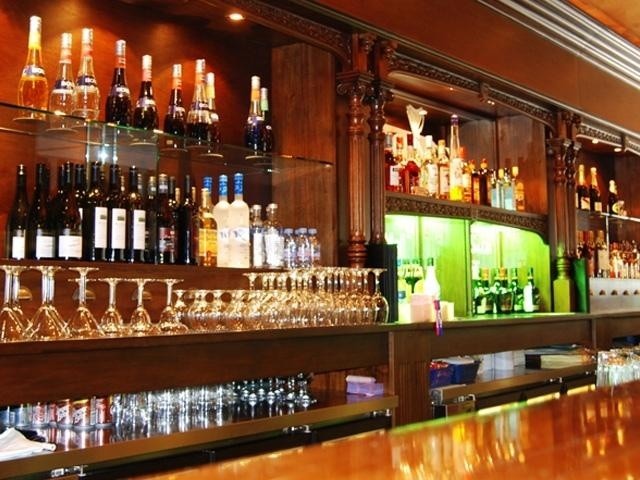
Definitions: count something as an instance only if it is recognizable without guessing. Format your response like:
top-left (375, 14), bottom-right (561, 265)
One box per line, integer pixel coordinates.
top-left (574, 165), bottom-right (591, 211)
top-left (576, 229), bottom-right (639, 279)
top-left (608, 180), bottom-right (619, 215)
top-left (7, 160), bottom-right (322, 267)
top-left (17, 14), bottom-right (276, 158)
top-left (384, 109), bottom-right (528, 213)
top-left (587, 167), bottom-right (603, 214)
top-left (470, 264), bottom-right (541, 314)
top-left (393, 256), bottom-right (444, 321)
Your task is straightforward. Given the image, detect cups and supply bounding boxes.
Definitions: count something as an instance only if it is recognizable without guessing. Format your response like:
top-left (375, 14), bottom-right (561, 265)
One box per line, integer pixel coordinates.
top-left (109, 372), bottom-right (315, 426)
top-left (441, 302), bottom-right (454, 321)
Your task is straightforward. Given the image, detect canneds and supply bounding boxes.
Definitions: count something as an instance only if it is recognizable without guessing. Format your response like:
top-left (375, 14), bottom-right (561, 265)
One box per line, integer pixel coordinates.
top-left (0, 392), bottom-right (114, 431)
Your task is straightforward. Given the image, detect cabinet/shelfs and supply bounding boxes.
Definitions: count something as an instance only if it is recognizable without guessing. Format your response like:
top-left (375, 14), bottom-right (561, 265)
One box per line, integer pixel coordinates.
top-left (0, 1), bottom-right (399, 480)
top-left (571, 106), bottom-right (639, 349)
top-left (374, 27), bottom-right (597, 430)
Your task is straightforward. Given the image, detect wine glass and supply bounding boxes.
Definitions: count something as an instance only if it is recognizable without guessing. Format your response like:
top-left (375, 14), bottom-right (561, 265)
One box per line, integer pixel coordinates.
top-left (0, 262), bottom-right (389, 345)
